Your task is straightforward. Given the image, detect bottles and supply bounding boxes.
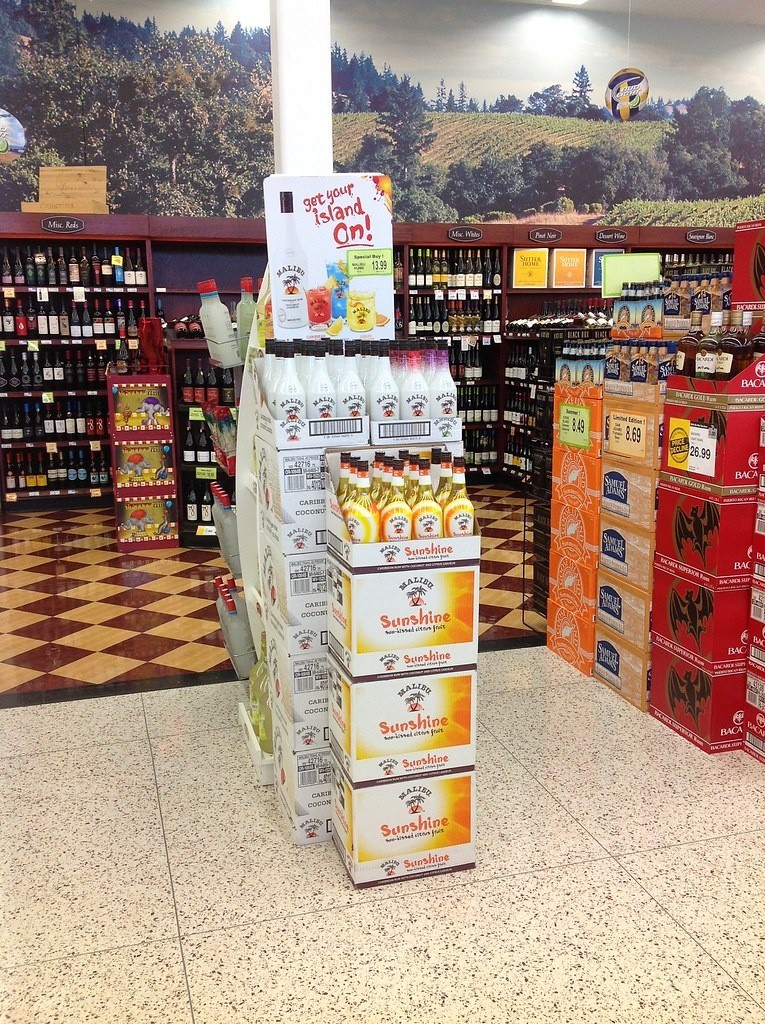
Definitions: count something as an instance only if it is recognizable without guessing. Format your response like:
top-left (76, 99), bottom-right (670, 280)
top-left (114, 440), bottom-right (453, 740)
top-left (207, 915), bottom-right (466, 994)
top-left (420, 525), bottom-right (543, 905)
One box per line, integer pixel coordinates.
top-left (258, 691), bottom-right (273, 754)
top-left (274, 191), bottom-right (309, 330)
top-left (252, 654), bottom-right (270, 736)
top-left (197, 281), bottom-right (218, 361)
top-left (0, 240), bottom-right (147, 484)
top-left (203, 278), bottom-right (241, 366)
top-left (182, 357), bottom-right (235, 405)
top-left (248, 631), bottom-right (267, 718)
top-left (263, 248), bottom-right (764, 543)
top-left (181, 420), bottom-right (218, 466)
top-left (158, 299), bottom-right (205, 338)
top-left (236, 278), bottom-right (248, 356)
top-left (238, 277), bottom-right (257, 361)
top-left (186, 478), bottom-right (257, 680)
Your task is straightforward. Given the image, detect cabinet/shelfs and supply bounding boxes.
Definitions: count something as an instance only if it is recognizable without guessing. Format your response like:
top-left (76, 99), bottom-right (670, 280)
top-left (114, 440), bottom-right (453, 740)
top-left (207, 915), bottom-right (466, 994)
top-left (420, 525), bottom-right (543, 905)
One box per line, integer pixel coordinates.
top-left (0, 213), bottom-right (735, 548)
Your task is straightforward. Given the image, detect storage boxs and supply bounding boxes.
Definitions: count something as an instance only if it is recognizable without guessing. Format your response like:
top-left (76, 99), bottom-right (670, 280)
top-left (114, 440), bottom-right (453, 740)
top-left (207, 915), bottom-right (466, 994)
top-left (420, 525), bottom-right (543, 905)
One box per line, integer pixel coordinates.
top-left (237, 362), bottom-right (484, 890)
top-left (20, 201), bottom-right (109, 214)
top-left (507, 212), bottom-right (765, 765)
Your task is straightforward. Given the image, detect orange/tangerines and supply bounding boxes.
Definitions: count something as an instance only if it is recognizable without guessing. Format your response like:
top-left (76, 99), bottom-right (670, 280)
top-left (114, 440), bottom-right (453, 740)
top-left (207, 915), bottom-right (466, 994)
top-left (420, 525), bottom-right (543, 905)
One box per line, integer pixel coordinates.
top-left (376, 313), bottom-right (390, 326)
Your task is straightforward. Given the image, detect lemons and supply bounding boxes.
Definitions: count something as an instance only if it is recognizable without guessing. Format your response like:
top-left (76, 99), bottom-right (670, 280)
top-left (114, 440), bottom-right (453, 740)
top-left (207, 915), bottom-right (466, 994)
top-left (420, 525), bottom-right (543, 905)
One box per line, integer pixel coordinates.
top-left (326, 316), bottom-right (343, 335)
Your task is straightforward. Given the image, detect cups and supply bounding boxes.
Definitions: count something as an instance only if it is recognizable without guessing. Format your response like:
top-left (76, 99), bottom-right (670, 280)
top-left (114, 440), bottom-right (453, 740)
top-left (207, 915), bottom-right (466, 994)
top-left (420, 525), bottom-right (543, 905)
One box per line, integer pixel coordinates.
top-left (345, 291), bottom-right (376, 332)
top-left (324, 262), bottom-right (351, 320)
top-left (303, 283), bottom-right (333, 332)
